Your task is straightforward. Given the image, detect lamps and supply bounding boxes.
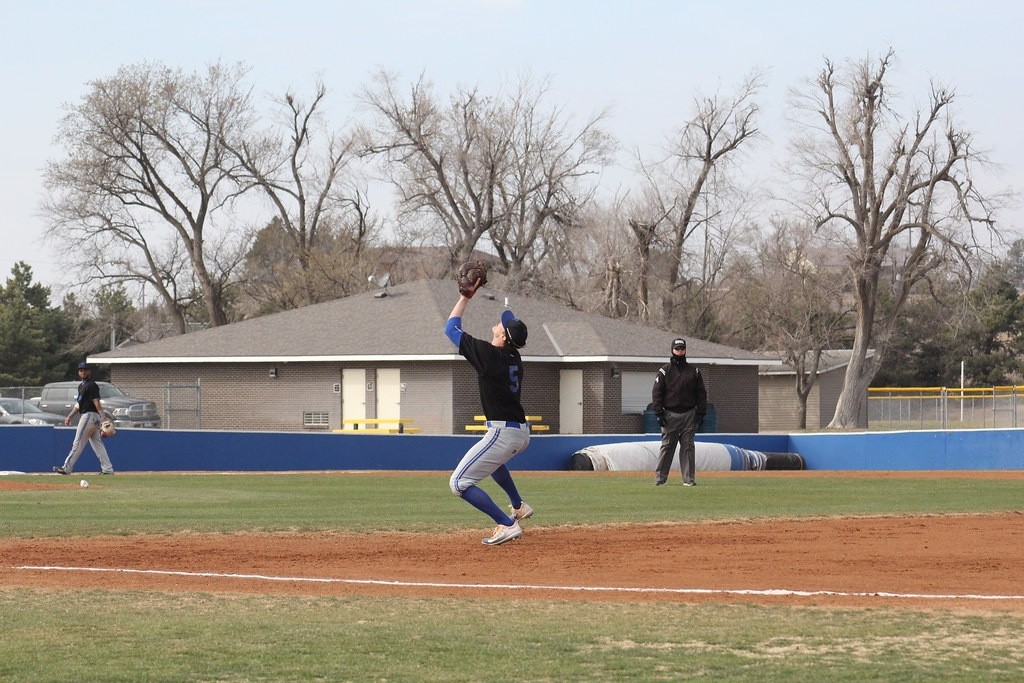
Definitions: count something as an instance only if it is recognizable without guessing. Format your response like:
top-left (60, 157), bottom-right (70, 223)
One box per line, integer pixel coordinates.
top-left (611, 368), bottom-right (620, 377)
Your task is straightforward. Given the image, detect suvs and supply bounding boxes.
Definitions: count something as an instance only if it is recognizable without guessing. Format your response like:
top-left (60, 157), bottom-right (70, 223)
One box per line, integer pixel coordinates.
top-left (38, 379), bottom-right (162, 430)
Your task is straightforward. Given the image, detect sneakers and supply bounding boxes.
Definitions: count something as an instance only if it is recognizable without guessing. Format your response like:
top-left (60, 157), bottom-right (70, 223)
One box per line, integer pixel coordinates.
top-left (482, 522), bottom-right (523, 546)
top-left (508, 501), bottom-right (533, 522)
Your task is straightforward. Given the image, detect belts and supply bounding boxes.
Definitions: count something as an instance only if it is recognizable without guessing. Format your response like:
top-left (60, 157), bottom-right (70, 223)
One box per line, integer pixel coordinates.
top-left (487, 421), bottom-right (527, 428)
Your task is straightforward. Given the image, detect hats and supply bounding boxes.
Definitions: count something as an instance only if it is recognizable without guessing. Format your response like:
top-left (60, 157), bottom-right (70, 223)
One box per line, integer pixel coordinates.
top-left (77, 362), bottom-right (90, 370)
top-left (501, 311), bottom-right (528, 348)
top-left (672, 339), bottom-right (686, 350)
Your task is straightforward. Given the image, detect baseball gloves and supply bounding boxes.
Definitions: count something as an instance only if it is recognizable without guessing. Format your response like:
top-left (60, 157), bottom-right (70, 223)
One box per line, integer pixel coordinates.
top-left (100, 420), bottom-right (117, 438)
top-left (457, 260), bottom-right (488, 299)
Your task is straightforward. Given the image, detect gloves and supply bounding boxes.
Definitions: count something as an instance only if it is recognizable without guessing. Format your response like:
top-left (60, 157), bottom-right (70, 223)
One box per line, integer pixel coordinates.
top-left (694, 414), bottom-right (703, 427)
top-left (657, 414), bottom-right (667, 427)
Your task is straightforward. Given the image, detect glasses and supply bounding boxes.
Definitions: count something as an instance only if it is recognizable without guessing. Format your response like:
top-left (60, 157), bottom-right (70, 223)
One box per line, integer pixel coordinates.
top-left (675, 346), bottom-right (686, 350)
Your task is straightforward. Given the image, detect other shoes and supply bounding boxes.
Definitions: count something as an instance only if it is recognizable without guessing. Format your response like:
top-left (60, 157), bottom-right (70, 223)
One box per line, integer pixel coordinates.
top-left (683, 481), bottom-right (696, 486)
top-left (53, 467), bottom-right (67, 474)
top-left (655, 481), bottom-right (667, 487)
top-left (100, 471), bottom-right (112, 475)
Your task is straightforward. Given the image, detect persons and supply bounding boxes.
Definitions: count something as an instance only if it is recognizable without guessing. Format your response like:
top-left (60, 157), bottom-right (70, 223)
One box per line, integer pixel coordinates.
top-left (57, 362), bottom-right (114, 475)
top-left (651, 338), bottom-right (707, 486)
top-left (444, 278), bottom-right (533, 546)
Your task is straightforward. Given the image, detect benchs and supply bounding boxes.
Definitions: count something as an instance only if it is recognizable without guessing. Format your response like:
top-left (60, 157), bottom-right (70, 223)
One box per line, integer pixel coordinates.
top-left (465, 416), bottom-right (549, 434)
top-left (333, 419), bottom-right (422, 434)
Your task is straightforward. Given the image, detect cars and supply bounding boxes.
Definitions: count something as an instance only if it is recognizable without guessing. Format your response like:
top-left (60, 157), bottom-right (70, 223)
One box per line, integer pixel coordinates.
top-left (0, 396), bottom-right (74, 428)
